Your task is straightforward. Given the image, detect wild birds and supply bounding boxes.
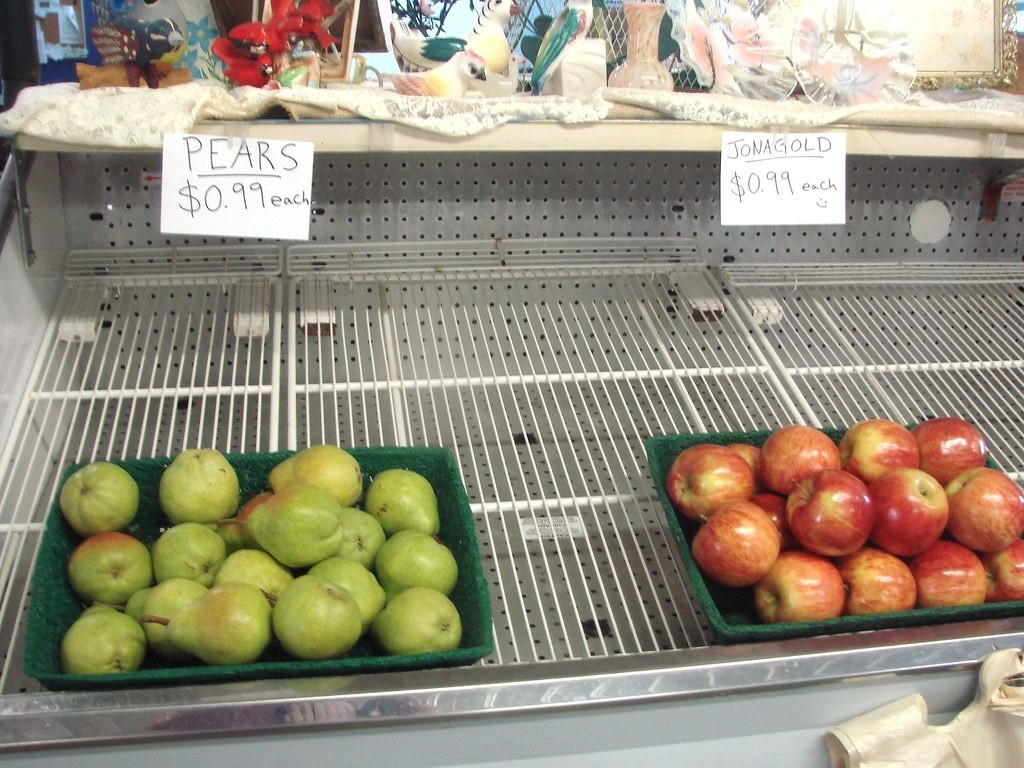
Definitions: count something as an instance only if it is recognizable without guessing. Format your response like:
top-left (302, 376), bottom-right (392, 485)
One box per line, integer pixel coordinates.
top-left (91, 14), bottom-right (186, 88)
top-left (379, 1), bottom-right (526, 98)
top-left (210, 0), bottom-right (341, 88)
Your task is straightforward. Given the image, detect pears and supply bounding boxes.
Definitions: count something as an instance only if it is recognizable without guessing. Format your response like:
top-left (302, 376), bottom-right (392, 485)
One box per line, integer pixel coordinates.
top-left (59, 440), bottom-right (465, 675)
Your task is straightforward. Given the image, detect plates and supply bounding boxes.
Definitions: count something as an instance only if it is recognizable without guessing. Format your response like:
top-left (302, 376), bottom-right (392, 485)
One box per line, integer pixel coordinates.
top-left (789, 0), bottom-right (917, 108)
top-left (665, 0), bottom-right (798, 99)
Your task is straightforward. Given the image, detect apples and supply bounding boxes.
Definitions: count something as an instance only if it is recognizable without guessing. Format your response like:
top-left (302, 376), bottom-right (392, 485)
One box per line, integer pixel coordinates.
top-left (667, 419), bottom-right (1023, 623)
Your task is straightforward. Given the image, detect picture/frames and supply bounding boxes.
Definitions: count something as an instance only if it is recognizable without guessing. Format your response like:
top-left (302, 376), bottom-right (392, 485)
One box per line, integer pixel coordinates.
top-left (773, 2), bottom-right (1020, 92)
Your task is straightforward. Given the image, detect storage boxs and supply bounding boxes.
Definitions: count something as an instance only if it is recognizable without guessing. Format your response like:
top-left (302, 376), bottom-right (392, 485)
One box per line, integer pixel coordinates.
top-left (20, 442), bottom-right (499, 689)
top-left (643, 421), bottom-right (1023, 638)
